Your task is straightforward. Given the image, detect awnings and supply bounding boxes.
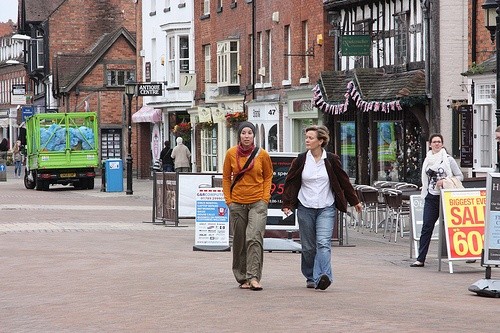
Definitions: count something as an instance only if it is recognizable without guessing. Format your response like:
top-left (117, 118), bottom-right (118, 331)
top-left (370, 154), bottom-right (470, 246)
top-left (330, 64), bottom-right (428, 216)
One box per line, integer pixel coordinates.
top-left (312, 69), bottom-right (427, 114)
top-left (131, 106), bottom-right (162, 124)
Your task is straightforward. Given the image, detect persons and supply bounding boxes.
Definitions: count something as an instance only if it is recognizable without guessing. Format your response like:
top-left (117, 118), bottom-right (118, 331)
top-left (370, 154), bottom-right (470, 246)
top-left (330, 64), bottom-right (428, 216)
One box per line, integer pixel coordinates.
top-left (223, 122), bottom-right (273, 291)
top-left (171, 137), bottom-right (191, 173)
top-left (160, 141), bottom-right (175, 172)
top-left (12, 141), bottom-right (22, 178)
top-left (281, 125), bottom-right (364, 291)
top-left (410, 133), bottom-right (476, 267)
top-left (0, 138), bottom-right (8, 161)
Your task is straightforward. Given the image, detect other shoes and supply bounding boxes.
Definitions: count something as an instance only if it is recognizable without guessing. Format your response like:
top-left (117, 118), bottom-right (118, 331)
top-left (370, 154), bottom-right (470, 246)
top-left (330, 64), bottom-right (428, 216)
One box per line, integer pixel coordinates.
top-left (240, 280), bottom-right (252, 289)
top-left (251, 277), bottom-right (263, 290)
top-left (306, 279), bottom-right (316, 288)
top-left (315, 274), bottom-right (331, 290)
top-left (410, 260), bottom-right (424, 267)
top-left (17, 176), bottom-right (21, 178)
top-left (466, 259), bottom-right (476, 263)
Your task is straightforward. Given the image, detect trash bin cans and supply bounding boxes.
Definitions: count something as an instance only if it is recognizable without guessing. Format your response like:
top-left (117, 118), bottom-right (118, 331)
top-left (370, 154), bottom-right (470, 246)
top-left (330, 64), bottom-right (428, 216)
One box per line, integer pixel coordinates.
top-left (101, 159), bottom-right (123, 192)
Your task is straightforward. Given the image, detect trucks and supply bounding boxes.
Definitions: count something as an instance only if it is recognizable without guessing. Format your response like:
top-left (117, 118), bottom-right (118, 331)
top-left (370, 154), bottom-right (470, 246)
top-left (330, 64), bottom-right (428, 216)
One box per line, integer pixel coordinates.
top-left (24, 112), bottom-right (99, 191)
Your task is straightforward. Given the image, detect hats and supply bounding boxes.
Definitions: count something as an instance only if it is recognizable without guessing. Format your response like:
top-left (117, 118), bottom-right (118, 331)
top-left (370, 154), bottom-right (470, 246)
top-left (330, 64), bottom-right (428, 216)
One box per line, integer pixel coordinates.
top-left (238, 121), bottom-right (256, 137)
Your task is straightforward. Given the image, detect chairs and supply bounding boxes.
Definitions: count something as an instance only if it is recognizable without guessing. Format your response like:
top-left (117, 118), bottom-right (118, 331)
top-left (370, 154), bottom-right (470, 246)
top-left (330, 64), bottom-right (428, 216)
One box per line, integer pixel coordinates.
top-left (347, 181), bottom-right (422, 242)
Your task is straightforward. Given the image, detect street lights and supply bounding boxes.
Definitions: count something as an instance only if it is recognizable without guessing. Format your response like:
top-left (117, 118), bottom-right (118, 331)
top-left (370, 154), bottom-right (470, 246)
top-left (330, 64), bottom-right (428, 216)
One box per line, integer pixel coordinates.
top-left (125, 78), bottom-right (136, 194)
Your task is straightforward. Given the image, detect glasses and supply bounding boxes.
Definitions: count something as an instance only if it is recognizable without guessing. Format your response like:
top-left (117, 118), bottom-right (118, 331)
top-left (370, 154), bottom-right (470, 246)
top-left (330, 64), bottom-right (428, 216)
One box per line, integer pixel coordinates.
top-left (431, 141), bottom-right (442, 143)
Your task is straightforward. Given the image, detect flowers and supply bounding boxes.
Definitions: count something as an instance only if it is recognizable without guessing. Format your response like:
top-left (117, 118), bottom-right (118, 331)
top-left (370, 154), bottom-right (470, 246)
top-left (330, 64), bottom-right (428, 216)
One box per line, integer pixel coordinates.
top-left (173, 123), bottom-right (190, 135)
top-left (194, 121), bottom-right (214, 136)
top-left (225, 112), bottom-right (247, 128)
